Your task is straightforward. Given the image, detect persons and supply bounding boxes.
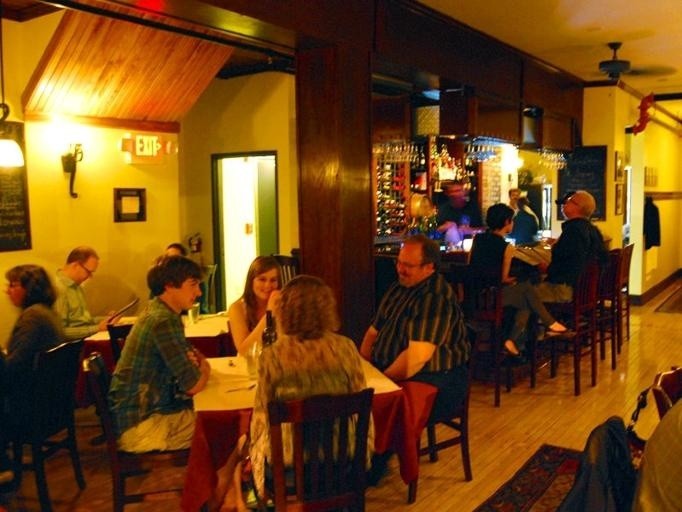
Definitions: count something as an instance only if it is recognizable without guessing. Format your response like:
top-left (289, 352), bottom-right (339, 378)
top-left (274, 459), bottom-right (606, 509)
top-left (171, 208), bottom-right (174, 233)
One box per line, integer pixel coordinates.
top-left (149, 243), bottom-right (210, 314)
top-left (0, 264), bottom-right (66, 491)
top-left (436, 180), bottom-right (616, 364)
top-left (201, 274), bottom-right (376, 511)
top-left (106, 255), bottom-right (211, 454)
top-left (359, 234), bottom-right (472, 485)
top-left (227, 255), bottom-right (286, 356)
top-left (51, 245), bottom-right (125, 342)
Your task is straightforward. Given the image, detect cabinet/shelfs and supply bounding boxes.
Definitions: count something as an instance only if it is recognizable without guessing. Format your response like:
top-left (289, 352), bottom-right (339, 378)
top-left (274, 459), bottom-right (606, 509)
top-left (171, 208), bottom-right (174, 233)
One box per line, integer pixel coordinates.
top-left (372, 142), bottom-right (411, 240)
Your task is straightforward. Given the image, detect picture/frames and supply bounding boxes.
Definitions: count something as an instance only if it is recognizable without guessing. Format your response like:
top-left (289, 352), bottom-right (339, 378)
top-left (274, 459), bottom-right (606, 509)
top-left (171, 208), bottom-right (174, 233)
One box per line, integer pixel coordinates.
top-left (614, 183), bottom-right (624, 217)
top-left (613, 151), bottom-right (627, 182)
top-left (112, 186), bottom-right (148, 224)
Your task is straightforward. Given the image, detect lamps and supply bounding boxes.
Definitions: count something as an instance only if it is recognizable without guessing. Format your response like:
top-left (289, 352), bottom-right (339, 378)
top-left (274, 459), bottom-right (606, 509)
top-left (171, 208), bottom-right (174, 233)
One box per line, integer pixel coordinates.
top-left (0, 102), bottom-right (27, 169)
top-left (55, 122), bottom-right (105, 199)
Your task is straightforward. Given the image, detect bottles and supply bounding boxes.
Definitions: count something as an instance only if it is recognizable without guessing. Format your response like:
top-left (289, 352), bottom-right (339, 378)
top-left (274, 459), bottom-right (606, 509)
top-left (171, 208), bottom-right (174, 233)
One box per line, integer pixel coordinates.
top-left (375, 165), bottom-right (407, 240)
top-left (260, 310), bottom-right (278, 346)
top-left (410, 165), bottom-right (428, 193)
top-left (431, 143), bottom-right (465, 181)
top-left (407, 213), bottom-right (438, 240)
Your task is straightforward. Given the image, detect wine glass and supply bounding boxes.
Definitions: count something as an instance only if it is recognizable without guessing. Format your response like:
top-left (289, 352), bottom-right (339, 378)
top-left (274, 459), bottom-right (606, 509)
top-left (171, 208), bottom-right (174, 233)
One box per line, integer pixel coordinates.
top-left (465, 138), bottom-right (499, 165)
top-left (535, 148), bottom-right (568, 169)
top-left (376, 143), bottom-right (425, 164)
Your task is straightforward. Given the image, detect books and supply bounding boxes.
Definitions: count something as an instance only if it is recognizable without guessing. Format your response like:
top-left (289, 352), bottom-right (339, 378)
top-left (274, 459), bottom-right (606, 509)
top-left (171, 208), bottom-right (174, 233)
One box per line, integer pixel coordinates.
top-left (108, 297), bottom-right (140, 322)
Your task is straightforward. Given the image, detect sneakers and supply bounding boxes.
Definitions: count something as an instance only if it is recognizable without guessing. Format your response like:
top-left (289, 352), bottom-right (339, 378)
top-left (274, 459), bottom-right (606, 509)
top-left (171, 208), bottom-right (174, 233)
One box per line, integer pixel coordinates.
top-left (544, 326), bottom-right (578, 337)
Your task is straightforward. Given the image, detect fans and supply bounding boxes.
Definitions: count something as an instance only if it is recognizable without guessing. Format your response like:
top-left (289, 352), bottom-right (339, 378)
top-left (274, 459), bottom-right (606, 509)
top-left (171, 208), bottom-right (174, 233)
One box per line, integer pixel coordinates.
top-left (547, 39), bottom-right (678, 82)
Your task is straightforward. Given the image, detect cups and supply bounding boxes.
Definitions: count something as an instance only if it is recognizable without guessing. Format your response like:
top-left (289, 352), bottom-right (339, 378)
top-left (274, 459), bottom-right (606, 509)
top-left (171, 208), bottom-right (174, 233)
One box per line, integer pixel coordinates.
top-left (245, 340), bottom-right (264, 381)
top-left (188, 302), bottom-right (200, 323)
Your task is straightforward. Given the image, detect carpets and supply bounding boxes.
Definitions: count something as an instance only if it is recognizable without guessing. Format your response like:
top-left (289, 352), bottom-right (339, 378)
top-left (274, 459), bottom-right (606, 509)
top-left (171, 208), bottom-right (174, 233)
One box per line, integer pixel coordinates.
top-left (652, 283), bottom-right (681, 315)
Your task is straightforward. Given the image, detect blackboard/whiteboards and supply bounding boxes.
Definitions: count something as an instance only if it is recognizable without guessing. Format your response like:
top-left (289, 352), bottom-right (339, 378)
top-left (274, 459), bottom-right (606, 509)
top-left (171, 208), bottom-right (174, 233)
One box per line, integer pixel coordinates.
top-left (0, 121), bottom-right (31, 251)
top-left (557, 144), bottom-right (610, 222)
